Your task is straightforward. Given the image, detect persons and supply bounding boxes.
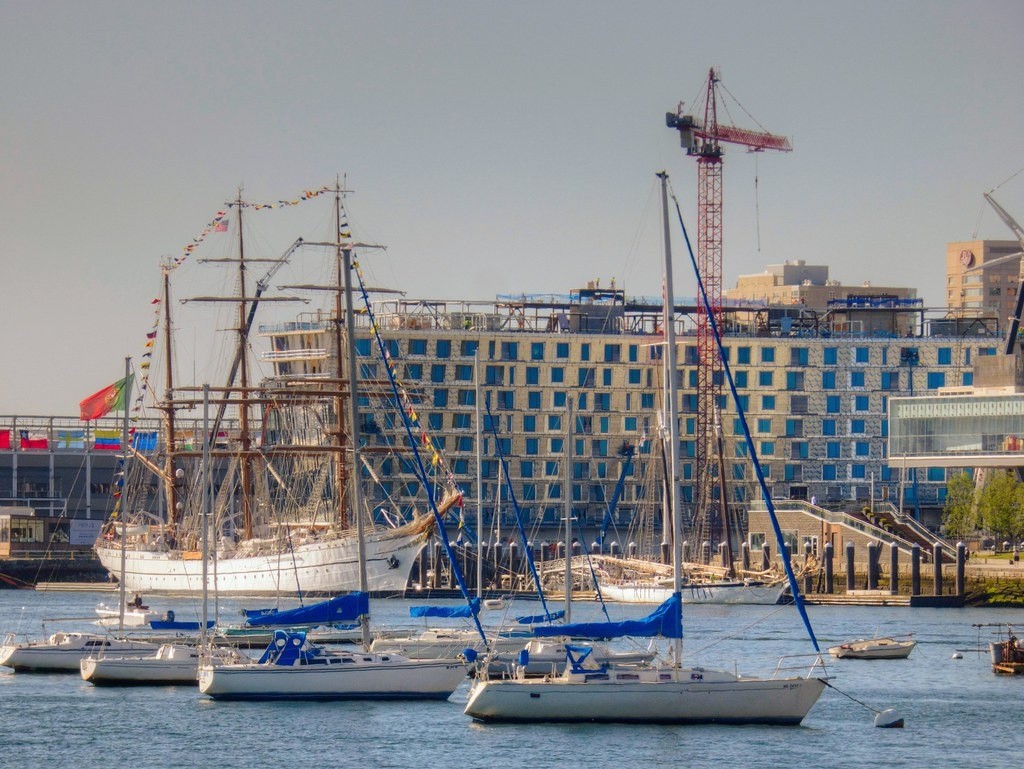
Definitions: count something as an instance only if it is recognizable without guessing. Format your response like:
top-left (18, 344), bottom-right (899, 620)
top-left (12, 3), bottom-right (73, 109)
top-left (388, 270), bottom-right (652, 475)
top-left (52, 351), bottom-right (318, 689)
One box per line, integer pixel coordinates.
top-left (965, 548), bottom-right (969, 566)
top-left (1014, 550), bottom-right (1019, 567)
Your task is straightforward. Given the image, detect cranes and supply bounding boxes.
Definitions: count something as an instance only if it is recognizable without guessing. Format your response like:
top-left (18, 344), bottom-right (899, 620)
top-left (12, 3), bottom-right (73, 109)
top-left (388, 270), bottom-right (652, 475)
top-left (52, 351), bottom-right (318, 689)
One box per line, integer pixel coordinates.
top-left (663, 61), bottom-right (794, 553)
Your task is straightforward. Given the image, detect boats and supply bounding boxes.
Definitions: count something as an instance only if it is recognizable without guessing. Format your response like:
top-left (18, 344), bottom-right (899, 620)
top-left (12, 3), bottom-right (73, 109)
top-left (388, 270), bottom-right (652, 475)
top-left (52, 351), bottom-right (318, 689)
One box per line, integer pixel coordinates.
top-left (826, 637), bottom-right (917, 662)
top-left (970, 619), bottom-right (1024, 677)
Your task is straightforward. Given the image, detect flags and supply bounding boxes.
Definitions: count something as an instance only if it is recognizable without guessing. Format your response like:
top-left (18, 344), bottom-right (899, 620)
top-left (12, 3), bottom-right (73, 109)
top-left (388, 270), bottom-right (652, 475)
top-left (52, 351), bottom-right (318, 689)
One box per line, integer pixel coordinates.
top-left (1, 186), bottom-right (465, 547)
top-left (80, 372), bottom-right (134, 421)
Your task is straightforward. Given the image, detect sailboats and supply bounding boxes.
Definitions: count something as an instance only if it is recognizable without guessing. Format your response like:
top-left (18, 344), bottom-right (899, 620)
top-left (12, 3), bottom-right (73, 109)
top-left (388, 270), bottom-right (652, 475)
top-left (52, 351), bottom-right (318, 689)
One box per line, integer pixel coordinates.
top-left (0, 169), bottom-right (842, 726)
